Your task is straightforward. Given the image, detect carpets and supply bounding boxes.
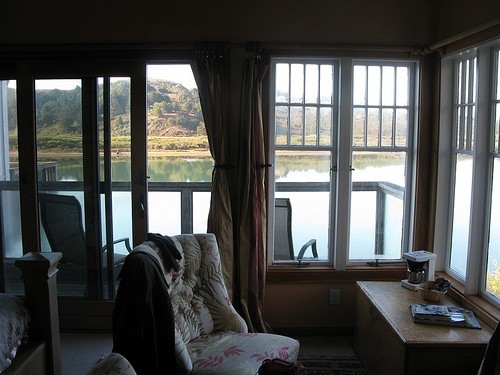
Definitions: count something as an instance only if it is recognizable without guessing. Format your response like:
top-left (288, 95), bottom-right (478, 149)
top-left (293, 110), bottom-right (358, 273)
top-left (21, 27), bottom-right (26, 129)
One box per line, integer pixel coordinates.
top-left (297, 355), bottom-right (367, 375)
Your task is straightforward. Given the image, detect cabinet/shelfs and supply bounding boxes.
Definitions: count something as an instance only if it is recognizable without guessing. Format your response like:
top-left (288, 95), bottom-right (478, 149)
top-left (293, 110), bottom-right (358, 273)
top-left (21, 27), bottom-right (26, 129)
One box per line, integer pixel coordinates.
top-left (353, 281), bottom-right (493, 375)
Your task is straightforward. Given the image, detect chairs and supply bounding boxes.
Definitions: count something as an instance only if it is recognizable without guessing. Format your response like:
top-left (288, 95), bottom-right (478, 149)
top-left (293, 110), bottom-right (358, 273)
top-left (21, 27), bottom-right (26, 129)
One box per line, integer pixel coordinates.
top-left (38, 192), bottom-right (133, 299)
top-left (112, 233), bottom-right (300, 375)
top-left (275, 198), bottom-right (318, 261)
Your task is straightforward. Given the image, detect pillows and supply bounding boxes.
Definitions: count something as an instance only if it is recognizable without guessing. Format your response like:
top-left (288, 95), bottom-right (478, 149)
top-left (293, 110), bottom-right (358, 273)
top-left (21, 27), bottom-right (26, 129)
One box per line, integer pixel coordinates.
top-left (0, 292), bottom-right (31, 375)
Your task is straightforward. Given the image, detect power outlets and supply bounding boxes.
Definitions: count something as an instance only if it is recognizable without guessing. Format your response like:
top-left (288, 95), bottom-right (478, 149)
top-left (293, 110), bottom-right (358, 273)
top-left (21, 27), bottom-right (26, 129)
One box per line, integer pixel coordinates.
top-left (329, 289), bottom-right (340, 304)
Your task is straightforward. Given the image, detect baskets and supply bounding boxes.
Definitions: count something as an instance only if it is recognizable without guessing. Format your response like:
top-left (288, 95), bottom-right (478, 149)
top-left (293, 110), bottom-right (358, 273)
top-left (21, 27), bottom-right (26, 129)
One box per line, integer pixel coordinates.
top-left (417, 284), bottom-right (447, 302)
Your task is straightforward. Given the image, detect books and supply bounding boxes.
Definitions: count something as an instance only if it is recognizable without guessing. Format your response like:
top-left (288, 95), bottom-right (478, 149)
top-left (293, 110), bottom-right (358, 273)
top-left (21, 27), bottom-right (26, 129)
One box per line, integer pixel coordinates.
top-left (409, 304), bottom-right (481, 329)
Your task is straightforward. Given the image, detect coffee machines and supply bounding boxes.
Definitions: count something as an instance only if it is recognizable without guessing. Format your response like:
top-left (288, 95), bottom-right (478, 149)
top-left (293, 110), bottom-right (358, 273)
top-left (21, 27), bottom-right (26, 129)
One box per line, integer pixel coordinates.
top-left (399, 250), bottom-right (437, 292)
